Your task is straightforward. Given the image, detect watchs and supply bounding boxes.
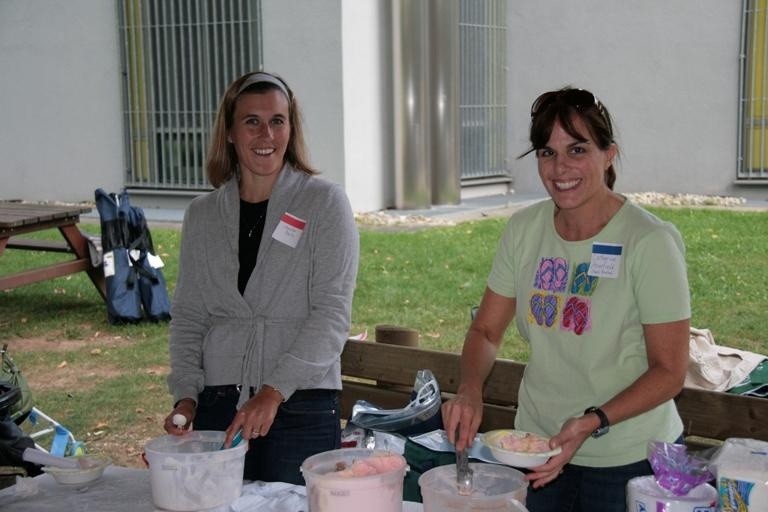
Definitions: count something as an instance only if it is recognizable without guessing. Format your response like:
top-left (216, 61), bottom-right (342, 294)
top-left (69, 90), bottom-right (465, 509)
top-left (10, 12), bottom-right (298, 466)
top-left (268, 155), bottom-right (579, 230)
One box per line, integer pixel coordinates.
top-left (582, 406), bottom-right (610, 439)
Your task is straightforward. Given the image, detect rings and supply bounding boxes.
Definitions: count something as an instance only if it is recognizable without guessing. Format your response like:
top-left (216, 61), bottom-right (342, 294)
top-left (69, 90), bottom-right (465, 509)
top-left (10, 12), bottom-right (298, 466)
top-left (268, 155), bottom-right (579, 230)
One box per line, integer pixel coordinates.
top-left (252, 432), bottom-right (260, 435)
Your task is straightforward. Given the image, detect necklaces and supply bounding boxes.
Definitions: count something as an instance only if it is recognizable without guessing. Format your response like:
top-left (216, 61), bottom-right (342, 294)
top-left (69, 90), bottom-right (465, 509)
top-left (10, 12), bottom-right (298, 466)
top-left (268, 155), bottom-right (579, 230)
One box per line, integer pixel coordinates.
top-left (239, 203), bottom-right (268, 238)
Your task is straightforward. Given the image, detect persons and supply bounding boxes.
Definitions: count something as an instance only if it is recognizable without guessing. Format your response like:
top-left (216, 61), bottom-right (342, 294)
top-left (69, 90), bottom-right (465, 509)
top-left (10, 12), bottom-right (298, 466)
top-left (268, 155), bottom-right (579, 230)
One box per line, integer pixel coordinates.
top-left (161, 70), bottom-right (359, 486)
top-left (440, 85), bottom-right (693, 512)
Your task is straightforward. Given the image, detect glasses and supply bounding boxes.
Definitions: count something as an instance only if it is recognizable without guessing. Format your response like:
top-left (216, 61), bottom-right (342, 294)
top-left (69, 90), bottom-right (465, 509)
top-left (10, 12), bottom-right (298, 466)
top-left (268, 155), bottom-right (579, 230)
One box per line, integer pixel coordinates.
top-left (530, 88), bottom-right (613, 141)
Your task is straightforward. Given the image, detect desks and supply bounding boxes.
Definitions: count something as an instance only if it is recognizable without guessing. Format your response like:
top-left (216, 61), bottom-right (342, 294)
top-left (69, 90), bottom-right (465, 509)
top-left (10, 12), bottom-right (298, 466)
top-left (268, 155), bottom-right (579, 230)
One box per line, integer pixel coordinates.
top-left (0, 201), bottom-right (108, 304)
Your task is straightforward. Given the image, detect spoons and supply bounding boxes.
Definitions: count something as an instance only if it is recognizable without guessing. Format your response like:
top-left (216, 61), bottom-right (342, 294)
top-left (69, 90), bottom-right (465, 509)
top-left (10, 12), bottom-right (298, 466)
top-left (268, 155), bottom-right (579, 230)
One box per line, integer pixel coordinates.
top-left (172, 414), bottom-right (187, 432)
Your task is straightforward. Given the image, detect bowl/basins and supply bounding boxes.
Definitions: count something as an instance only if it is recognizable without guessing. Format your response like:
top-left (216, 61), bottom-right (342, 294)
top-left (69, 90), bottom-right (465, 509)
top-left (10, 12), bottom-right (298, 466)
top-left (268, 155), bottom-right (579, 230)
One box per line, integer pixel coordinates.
top-left (479, 429), bottom-right (563, 469)
top-left (40, 453), bottom-right (113, 486)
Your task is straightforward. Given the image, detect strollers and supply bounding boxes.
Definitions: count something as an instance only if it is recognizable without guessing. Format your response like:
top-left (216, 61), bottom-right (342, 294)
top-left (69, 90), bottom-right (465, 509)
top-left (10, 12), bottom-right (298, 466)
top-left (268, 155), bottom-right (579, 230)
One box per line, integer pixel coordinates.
top-left (0, 345), bottom-right (84, 486)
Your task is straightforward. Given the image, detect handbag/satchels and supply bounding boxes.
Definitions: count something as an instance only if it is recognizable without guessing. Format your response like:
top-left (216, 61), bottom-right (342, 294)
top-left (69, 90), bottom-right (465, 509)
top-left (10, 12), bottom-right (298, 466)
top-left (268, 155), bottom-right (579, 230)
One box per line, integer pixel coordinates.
top-left (345, 368), bottom-right (442, 437)
top-left (94, 188), bottom-right (171, 325)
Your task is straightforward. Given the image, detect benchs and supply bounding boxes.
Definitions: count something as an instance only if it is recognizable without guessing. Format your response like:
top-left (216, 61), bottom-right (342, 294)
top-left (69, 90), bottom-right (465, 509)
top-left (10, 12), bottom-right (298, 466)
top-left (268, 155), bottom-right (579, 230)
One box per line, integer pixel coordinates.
top-left (337, 324), bottom-right (768, 452)
